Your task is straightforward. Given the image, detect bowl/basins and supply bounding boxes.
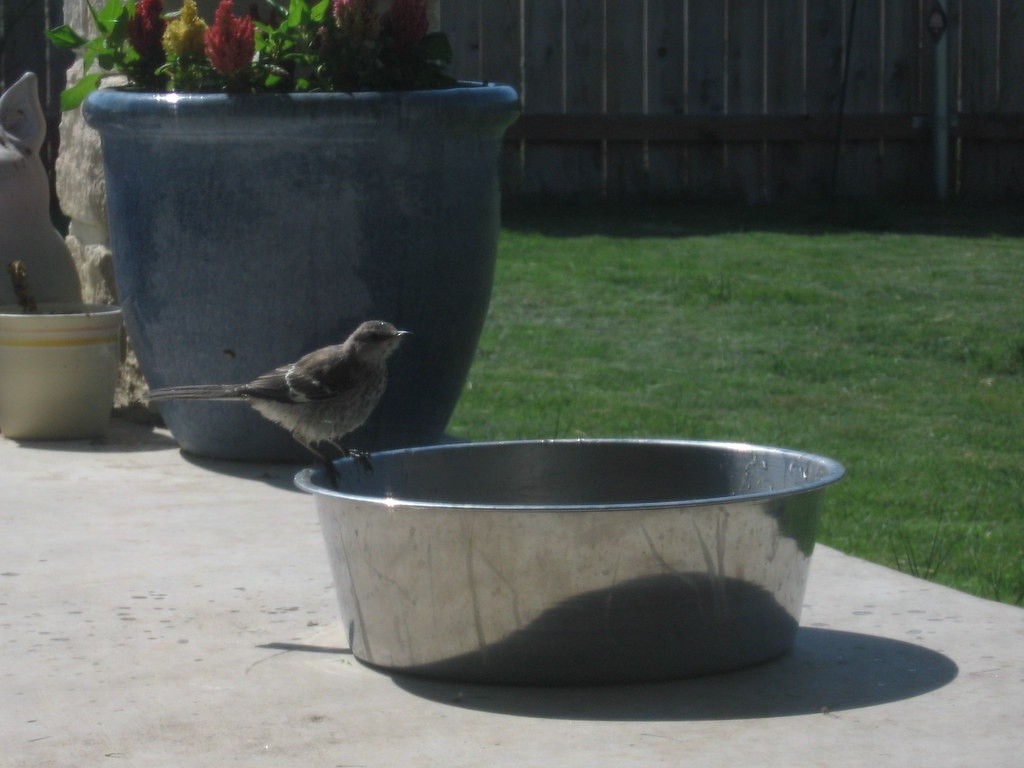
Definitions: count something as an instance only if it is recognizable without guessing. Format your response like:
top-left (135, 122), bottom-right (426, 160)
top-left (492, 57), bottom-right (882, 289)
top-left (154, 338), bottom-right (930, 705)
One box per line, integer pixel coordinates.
top-left (292, 437), bottom-right (848, 687)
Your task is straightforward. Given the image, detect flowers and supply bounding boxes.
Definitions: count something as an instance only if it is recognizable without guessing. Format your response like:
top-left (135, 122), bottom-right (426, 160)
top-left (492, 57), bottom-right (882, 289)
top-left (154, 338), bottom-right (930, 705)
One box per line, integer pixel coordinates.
top-left (41, 0), bottom-right (461, 111)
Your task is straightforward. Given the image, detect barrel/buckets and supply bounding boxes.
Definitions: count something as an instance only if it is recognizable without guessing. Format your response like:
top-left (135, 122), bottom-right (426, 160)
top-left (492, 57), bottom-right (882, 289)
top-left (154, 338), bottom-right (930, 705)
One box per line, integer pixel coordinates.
top-left (0, 302), bottom-right (121, 440)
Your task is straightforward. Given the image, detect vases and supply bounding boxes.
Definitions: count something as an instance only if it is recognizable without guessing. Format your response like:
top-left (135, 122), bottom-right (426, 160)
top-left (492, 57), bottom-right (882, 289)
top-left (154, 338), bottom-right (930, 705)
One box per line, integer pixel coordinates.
top-left (0, 302), bottom-right (124, 441)
top-left (82, 78), bottom-right (521, 464)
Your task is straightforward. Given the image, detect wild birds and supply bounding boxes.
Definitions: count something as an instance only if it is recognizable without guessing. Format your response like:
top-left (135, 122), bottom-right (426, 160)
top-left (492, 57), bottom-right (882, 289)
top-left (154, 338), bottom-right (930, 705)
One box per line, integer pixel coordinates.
top-left (139, 320), bottom-right (415, 490)
